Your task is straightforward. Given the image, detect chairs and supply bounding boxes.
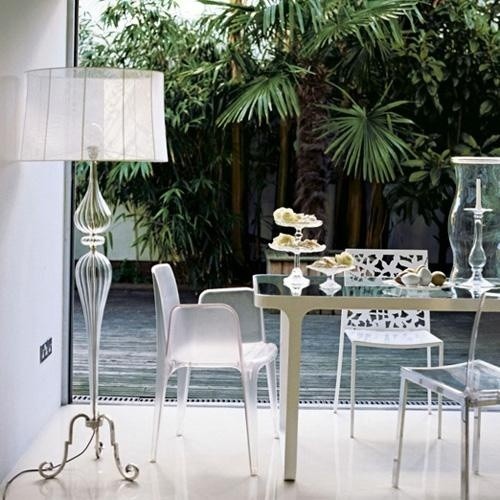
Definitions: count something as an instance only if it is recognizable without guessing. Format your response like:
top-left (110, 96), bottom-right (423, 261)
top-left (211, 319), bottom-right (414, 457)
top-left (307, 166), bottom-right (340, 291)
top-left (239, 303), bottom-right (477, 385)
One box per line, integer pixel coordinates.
top-left (151, 263), bottom-right (280, 477)
top-left (394, 289), bottom-right (498, 500)
top-left (331, 245), bottom-right (444, 446)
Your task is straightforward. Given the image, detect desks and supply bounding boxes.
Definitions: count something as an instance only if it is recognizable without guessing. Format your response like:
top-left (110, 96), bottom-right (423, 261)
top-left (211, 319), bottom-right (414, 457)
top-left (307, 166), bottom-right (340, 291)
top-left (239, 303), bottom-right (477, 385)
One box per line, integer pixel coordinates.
top-left (251, 272), bottom-right (500, 486)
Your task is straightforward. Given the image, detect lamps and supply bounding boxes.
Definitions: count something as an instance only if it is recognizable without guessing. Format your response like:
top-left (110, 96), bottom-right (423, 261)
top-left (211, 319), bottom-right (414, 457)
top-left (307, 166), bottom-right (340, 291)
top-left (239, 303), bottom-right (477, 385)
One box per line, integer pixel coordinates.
top-left (17, 65), bottom-right (170, 484)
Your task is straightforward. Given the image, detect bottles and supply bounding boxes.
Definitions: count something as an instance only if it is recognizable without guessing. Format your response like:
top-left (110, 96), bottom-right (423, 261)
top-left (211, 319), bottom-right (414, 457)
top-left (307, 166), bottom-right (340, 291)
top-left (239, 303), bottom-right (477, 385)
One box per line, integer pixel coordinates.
top-left (447, 156), bottom-right (500, 288)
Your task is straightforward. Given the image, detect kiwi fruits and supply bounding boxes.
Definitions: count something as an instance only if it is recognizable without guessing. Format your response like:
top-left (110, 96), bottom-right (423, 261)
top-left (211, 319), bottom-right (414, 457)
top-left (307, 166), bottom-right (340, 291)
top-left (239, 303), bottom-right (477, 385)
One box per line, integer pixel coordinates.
top-left (395, 265), bottom-right (445, 285)
